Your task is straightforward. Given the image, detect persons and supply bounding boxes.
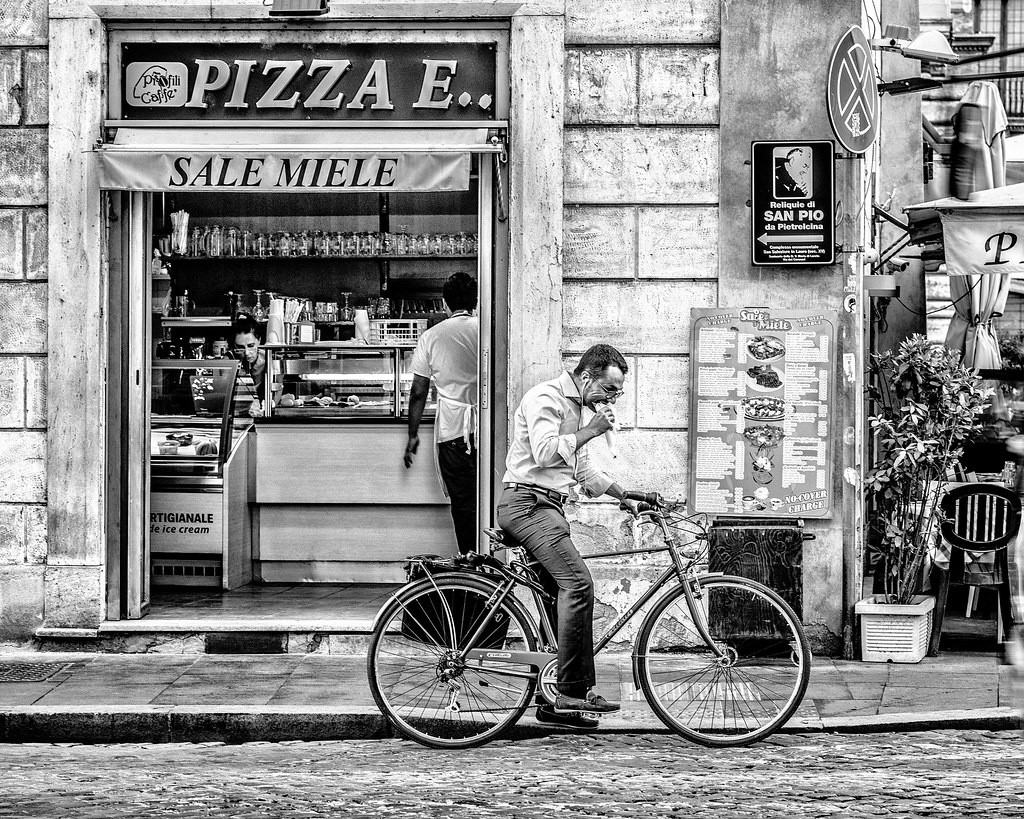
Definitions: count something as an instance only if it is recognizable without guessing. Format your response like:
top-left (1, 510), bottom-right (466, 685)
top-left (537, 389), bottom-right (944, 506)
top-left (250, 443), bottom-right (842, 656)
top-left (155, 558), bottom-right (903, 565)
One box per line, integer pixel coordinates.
top-left (222, 318), bottom-right (266, 390)
top-left (775, 148), bottom-right (811, 198)
top-left (496, 344), bottom-right (643, 729)
top-left (403, 270), bottom-right (479, 555)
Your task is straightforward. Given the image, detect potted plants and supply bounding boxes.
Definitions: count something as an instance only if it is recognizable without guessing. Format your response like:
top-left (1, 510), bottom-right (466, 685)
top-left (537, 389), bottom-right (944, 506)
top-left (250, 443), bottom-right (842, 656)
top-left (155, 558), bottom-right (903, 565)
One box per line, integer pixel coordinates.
top-left (854, 332), bottom-right (997, 662)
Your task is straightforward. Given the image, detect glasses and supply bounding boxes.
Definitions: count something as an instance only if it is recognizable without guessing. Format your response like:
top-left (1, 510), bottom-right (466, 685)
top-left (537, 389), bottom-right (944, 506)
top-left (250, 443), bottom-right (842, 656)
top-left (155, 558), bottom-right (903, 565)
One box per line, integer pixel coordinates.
top-left (587, 371), bottom-right (625, 398)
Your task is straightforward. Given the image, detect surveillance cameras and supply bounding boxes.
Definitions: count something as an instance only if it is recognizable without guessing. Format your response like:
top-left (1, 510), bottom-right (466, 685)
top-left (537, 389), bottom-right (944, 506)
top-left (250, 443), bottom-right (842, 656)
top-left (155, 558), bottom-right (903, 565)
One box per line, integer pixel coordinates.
top-left (887, 257), bottom-right (910, 273)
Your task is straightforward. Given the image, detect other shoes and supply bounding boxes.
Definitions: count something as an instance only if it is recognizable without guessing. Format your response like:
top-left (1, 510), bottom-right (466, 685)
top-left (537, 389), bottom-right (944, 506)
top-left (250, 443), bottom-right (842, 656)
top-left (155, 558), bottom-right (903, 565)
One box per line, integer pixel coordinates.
top-left (554, 689), bottom-right (621, 714)
top-left (534, 706), bottom-right (599, 730)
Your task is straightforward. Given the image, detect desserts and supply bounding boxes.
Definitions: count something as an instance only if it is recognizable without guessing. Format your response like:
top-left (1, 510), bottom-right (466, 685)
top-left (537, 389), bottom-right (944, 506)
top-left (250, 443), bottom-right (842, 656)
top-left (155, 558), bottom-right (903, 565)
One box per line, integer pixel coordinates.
top-left (158, 433), bottom-right (217, 456)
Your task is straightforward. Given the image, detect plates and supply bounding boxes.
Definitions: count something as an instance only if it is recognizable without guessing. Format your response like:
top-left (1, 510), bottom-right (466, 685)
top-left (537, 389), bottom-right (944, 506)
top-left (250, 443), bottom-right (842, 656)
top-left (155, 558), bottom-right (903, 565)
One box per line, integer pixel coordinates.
top-left (745, 335), bottom-right (786, 363)
top-left (746, 366), bottom-right (785, 393)
top-left (734, 396), bottom-right (796, 421)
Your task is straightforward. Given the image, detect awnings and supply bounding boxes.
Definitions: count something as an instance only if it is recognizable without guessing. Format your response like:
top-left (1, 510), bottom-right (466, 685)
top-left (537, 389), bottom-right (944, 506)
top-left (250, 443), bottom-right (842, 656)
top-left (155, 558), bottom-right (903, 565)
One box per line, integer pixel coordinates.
top-left (92, 127), bottom-right (504, 193)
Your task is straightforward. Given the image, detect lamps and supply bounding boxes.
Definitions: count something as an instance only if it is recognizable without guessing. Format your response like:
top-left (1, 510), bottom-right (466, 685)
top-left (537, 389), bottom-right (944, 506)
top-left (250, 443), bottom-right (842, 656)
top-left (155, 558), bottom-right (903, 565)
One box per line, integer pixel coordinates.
top-left (877, 75), bottom-right (944, 97)
top-left (946, 20), bottom-right (995, 52)
top-left (869, 31), bottom-right (960, 65)
top-left (922, 99), bottom-right (985, 202)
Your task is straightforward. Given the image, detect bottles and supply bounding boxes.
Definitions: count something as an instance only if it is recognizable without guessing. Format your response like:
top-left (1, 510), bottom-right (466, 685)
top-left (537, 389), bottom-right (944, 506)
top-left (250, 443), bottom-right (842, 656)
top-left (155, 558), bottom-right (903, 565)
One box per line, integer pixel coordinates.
top-left (299, 312), bottom-right (315, 344)
top-left (226, 227), bottom-right (480, 256)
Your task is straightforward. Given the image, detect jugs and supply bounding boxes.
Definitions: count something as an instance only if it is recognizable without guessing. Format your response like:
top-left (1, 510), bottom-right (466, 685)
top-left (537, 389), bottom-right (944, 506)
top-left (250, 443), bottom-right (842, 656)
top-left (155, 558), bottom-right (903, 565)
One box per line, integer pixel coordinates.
top-left (187, 225), bottom-right (226, 257)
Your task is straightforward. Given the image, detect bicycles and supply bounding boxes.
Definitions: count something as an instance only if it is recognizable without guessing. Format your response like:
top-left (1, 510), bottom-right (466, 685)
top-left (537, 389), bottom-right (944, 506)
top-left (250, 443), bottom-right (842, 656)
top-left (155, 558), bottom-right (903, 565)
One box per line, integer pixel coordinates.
top-left (364, 487), bottom-right (811, 751)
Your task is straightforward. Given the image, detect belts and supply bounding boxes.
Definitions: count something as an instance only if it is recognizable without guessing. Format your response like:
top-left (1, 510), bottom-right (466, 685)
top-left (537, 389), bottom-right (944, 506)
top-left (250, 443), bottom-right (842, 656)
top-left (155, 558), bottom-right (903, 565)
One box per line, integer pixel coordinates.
top-left (503, 482), bottom-right (566, 504)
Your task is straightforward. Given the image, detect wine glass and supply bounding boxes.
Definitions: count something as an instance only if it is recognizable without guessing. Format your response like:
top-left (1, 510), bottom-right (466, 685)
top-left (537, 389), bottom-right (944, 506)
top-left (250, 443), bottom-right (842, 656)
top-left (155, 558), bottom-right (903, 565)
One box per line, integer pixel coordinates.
top-left (234, 294), bottom-right (245, 319)
top-left (341, 292), bottom-right (352, 320)
top-left (253, 290), bottom-right (266, 319)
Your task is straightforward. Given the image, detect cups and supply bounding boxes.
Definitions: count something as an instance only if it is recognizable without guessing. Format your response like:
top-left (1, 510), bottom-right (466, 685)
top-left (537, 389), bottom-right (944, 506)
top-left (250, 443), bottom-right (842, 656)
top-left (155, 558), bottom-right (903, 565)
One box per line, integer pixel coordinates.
top-left (770, 498), bottom-right (782, 510)
top-left (176, 296), bottom-right (186, 317)
top-left (742, 496), bottom-right (755, 507)
